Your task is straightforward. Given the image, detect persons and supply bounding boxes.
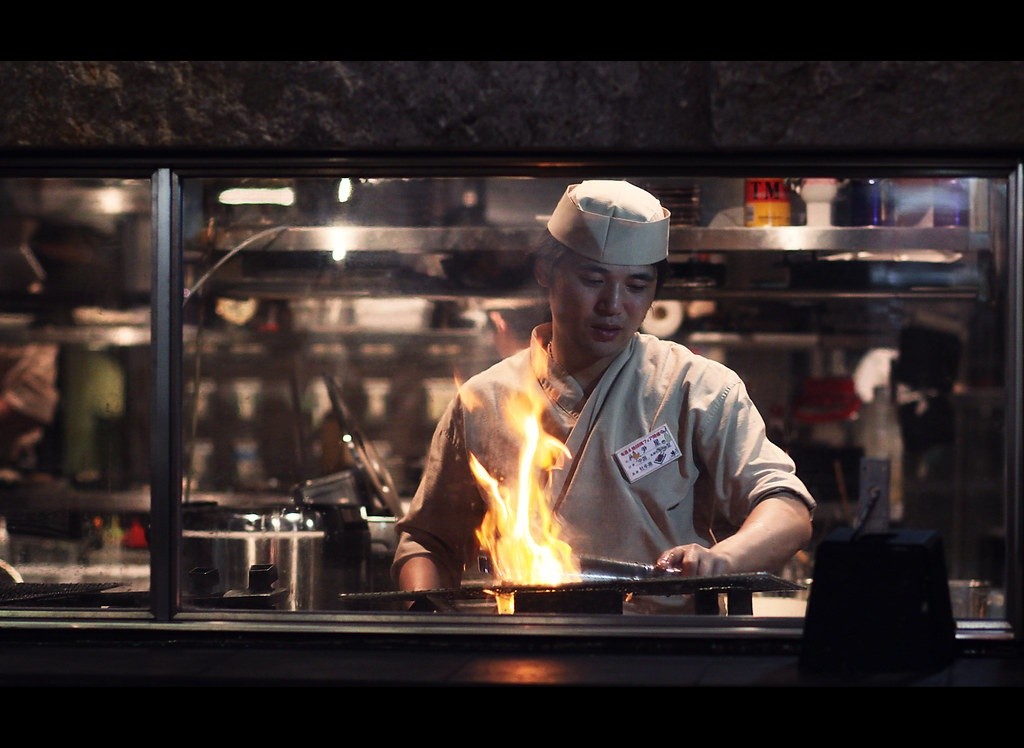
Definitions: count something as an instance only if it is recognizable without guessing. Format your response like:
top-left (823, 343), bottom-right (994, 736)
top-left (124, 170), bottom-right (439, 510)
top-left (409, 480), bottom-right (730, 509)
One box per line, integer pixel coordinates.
top-left (0, 345), bottom-right (59, 481)
top-left (392, 181), bottom-right (815, 616)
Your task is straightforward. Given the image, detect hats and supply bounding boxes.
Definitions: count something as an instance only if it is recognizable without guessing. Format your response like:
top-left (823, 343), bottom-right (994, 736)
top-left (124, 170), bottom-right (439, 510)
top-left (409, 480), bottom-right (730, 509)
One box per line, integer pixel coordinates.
top-left (547, 180), bottom-right (671, 266)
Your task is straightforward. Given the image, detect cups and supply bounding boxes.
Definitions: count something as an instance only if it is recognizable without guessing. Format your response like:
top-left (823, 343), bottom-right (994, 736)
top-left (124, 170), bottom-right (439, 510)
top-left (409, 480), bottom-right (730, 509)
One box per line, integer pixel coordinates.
top-left (933, 177), bottom-right (969, 228)
top-left (848, 180), bottom-right (880, 224)
top-left (744, 178), bottom-right (792, 227)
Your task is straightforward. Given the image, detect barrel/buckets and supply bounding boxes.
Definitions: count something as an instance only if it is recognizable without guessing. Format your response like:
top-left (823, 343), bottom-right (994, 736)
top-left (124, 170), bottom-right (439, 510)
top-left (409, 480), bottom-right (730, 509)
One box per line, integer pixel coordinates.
top-left (317, 517), bottom-right (410, 613)
top-left (182, 528), bottom-right (327, 613)
